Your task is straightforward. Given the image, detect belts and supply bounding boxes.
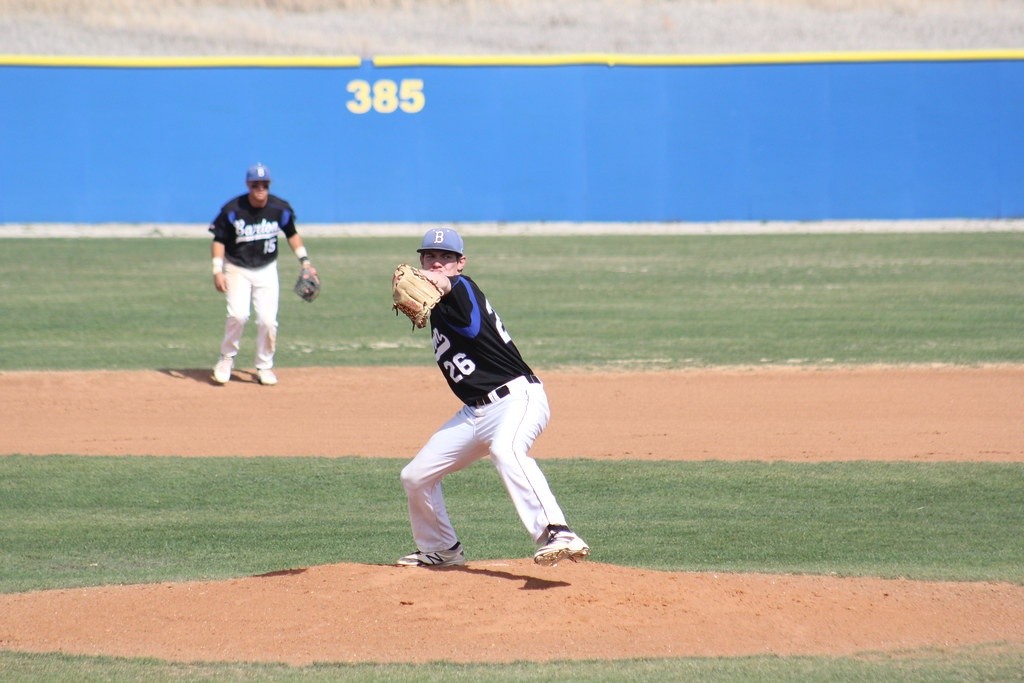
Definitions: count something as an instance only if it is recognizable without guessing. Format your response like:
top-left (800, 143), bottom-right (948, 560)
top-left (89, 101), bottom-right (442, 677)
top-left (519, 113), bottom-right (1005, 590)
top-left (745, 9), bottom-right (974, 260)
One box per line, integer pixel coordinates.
top-left (475, 374), bottom-right (539, 408)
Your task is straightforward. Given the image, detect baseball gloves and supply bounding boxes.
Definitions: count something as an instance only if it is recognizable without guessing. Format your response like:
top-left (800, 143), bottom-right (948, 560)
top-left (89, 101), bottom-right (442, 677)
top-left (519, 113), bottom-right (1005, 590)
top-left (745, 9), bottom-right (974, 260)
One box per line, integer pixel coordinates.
top-left (392, 263), bottom-right (445, 329)
top-left (292, 264), bottom-right (322, 303)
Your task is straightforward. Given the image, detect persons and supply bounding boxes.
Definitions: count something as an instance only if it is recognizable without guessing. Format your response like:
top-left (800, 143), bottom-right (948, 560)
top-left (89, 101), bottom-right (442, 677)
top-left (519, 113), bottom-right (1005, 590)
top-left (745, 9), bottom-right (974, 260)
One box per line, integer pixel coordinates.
top-left (208, 164), bottom-right (320, 385)
top-left (392, 228), bottom-right (591, 566)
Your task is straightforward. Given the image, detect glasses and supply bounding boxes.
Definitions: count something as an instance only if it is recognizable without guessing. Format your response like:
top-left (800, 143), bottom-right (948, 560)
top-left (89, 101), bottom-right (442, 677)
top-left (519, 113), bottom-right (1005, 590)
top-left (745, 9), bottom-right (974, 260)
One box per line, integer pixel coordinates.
top-left (250, 184), bottom-right (269, 189)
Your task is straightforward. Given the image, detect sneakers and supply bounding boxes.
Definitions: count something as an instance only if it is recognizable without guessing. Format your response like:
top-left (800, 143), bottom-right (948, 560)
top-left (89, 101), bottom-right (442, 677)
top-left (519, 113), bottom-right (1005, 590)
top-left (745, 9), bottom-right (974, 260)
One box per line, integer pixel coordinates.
top-left (214, 351), bottom-right (234, 383)
top-left (257, 366), bottom-right (277, 384)
top-left (396, 540), bottom-right (465, 568)
top-left (534, 530), bottom-right (590, 565)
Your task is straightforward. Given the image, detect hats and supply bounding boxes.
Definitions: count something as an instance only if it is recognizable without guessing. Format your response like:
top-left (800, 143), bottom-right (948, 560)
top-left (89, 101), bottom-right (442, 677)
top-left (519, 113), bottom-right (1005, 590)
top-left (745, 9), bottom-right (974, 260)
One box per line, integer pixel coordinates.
top-left (416, 227), bottom-right (464, 255)
top-left (247, 164), bottom-right (271, 181)
